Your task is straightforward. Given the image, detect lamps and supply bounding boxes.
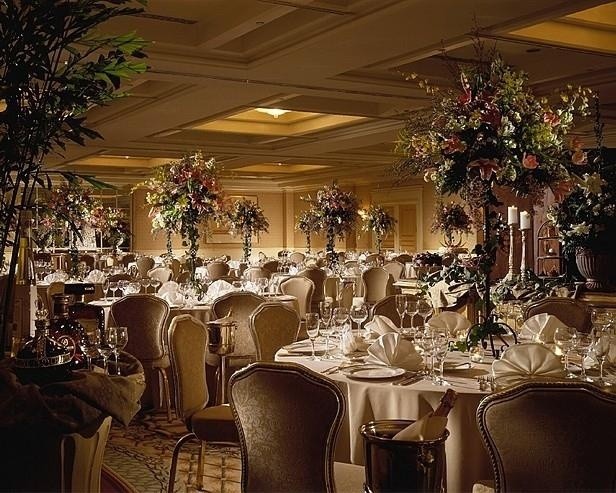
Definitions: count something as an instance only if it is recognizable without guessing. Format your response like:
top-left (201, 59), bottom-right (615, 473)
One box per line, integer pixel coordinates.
top-left (253, 107), bottom-right (291, 118)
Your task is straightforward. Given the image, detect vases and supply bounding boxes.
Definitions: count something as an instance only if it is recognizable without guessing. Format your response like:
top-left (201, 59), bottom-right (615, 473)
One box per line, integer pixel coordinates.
top-left (572, 248), bottom-right (616, 290)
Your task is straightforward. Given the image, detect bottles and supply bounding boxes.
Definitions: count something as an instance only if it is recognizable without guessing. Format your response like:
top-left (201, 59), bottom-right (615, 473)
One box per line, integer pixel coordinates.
top-left (46, 292), bottom-right (91, 370)
top-left (65, 282), bottom-right (116, 368)
top-left (393, 388), bottom-right (460, 441)
top-left (15, 308), bottom-right (73, 384)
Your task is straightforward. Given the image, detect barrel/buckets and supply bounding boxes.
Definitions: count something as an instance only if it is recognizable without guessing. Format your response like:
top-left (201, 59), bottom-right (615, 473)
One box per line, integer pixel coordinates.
top-left (360, 419), bottom-right (450, 493)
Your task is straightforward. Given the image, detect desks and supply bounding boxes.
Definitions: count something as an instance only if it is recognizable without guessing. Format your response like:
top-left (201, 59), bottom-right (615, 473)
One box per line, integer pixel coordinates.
top-left (391, 278), bottom-right (615, 330)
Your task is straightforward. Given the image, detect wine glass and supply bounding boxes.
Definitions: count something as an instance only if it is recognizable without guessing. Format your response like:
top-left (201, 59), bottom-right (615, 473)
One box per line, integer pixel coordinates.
top-left (109, 281), bottom-right (119, 301)
top-left (271, 272), bottom-right (281, 299)
top-left (395, 294), bottom-right (408, 331)
top-left (104, 263), bottom-right (138, 276)
top-left (414, 327), bottom-right (450, 386)
top-left (78, 329), bottom-right (100, 371)
top-left (405, 301), bottom-right (419, 331)
top-left (232, 282), bottom-right (244, 290)
top-left (593, 329), bottom-right (613, 385)
top-left (333, 306), bottom-right (351, 359)
top-left (142, 278), bottom-right (151, 293)
top-left (177, 282), bottom-right (197, 309)
top-left (591, 307), bottom-right (616, 332)
top-left (150, 278), bottom-right (160, 295)
top-left (416, 299), bottom-right (433, 326)
top-left (349, 304), bottom-right (368, 336)
top-left (320, 301), bottom-right (332, 332)
top-left (255, 277), bottom-right (269, 296)
top-left (106, 326), bottom-right (128, 374)
top-left (277, 257), bottom-right (304, 274)
top-left (101, 278), bottom-right (109, 304)
top-left (319, 317), bottom-right (335, 360)
top-left (571, 333), bottom-right (594, 381)
top-left (324, 261), bottom-right (352, 277)
top-left (553, 326), bottom-right (579, 379)
top-left (119, 279), bottom-right (141, 295)
top-left (306, 313), bottom-right (320, 361)
top-left (492, 299), bottom-right (524, 335)
top-left (95, 329), bottom-right (117, 374)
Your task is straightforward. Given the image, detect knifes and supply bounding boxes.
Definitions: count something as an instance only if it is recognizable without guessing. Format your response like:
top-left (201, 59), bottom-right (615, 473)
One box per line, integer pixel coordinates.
top-left (391, 371), bottom-right (425, 385)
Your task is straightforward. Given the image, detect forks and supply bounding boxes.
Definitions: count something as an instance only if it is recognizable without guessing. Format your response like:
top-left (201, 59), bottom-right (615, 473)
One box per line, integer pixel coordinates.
top-left (320, 360), bottom-right (346, 375)
top-left (477, 374), bottom-right (496, 391)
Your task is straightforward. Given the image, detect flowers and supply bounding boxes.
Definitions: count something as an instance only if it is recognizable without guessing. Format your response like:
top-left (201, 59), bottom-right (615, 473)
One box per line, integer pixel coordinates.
top-left (93, 206), bottom-right (134, 257)
top-left (296, 182), bottom-right (363, 261)
top-left (9, 200), bottom-right (64, 250)
top-left (46, 178), bottom-right (104, 277)
top-left (428, 202), bottom-right (474, 243)
top-left (386, 7), bottom-right (608, 353)
top-left (292, 209), bottom-right (323, 252)
top-left (227, 196), bottom-right (271, 264)
top-left (129, 163), bottom-right (181, 257)
top-left (411, 251), bottom-right (442, 267)
top-left (547, 91), bottom-right (616, 253)
top-left (356, 199), bottom-right (397, 249)
top-left (128, 149), bottom-right (233, 297)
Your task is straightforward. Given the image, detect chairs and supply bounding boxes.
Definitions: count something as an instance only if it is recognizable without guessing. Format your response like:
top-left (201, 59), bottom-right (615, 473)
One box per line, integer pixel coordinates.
top-left (171, 258), bottom-right (180, 279)
top-left (395, 254), bottom-right (412, 263)
top-left (239, 263), bottom-right (246, 274)
top-left (45, 280), bottom-right (64, 319)
top-left (159, 251), bottom-right (172, 258)
top-left (205, 291), bottom-right (265, 406)
top-left (105, 254), bottom-right (118, 266)
top-left (472, 379), bottom-right (616, 492)
top-left (365, 253), bottom-right (383, 265)
top-left (137, 257), bottom-right (154, 278)
top-left (103, 274), bottom-right (131, 296)
top-left (279, 276), bottom-right (315, 323)
top-left (381, 260), bottom-right (404, 280)
top-left (360, 267), bottom-right (389, 318)
top-left (175, 271), bottom-right (190, 282)
top-left (523, 296), bottom-right (592, 332)
top-left (345, 248), bottom-right (356, 254)
top-left (209, 275), bottom-right (242, 290)
top-left (263, 260), bottom-right (278, 271)
top-left (287, 251), bottom-right (304, 263)
top-left (278, 249), bottom-right (290, 256)
top-left (225, 362), bottom-right (366, 493)
top-left (316, 256), bottom-right (327, 266)
top-left (194, 256), bottom-right (205, 266)
top-left (80, 254), bottom-right (94, 270)
top-left (337, 251), bottom-right (345, 264)
top-left (206, 261), bottom-right (229, 279)
top-left (248, 302), bottom-right (300, 361)
top-left (242, 266), bottom-right (270, 291)
top-left (108, 294), bottom-right (172, 423)
top-left (297, 267), bottom-right (326, 318)
top-left (167, 314), bottom-right (240, 491)
top-left (440, 252), bottom-right (454, 265)
top-left (371, 293), bottom-right (433, 327)
top-left (122, 252), bottom-right (134, 266)
top-left (258, 251), bottom-right (266, 259)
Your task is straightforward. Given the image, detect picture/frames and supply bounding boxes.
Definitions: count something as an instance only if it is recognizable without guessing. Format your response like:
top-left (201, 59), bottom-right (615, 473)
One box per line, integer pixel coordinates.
top-left (203, 194), bottom-right (258, 243)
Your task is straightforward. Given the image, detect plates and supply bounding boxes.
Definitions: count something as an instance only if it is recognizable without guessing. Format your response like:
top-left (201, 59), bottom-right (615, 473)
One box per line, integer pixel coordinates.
top-left (287, 341), bottom-right (337, 352)
top-left (339, 363), bottom-right (406, 381)
top-left (363, 338), bottom-right (379, 345)
top-left (194, 302), bottom-right (207, 306)
top-left (433, 361), bottom-right (477, 376)
top-left (260, 292), bottom-right (283, 297)
top-left (277, 295), bottom-right (298, 302)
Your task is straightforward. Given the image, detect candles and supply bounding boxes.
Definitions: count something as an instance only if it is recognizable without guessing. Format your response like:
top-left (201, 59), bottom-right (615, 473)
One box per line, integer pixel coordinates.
top-left (507, 204), bottom-right (518, 224)
top-left (519, 210), bottom-right (531, 228)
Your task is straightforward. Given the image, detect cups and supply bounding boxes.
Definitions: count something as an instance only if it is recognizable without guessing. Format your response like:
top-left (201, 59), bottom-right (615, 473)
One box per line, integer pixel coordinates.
top-left (468, 346), bottom-right (485, 362)
